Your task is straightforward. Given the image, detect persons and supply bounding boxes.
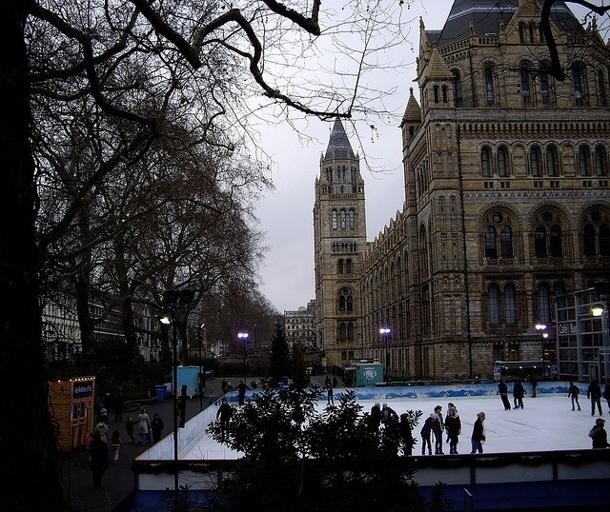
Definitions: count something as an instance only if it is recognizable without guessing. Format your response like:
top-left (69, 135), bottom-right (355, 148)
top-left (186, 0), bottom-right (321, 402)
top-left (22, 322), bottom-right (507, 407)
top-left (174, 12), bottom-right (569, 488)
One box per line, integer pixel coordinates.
top-left (568, 381), bottom-right (581, 410)
top-left (249, 380), bottom-right (257, 389)
top-left (322, 372), bottom-right (356, 405)
top-left (446, 403), bottom-right (458, 449)
top-left (603, 377), bottom-right (610, 414)
top-left (216, 397), bottom-right (232, 443)
top-left (433, 406), bottom-right (445, 454)
top-left (88, 385), bottom-right (164, 488)
top-left (236, 380), bottom-right (251, 405)
top-left (222, 379), bottom-right (227, 393)
top-left (498, 374), bottom-right (537, 409)
top-left (589, 418), bottom-right (610, 448)
top-left (176, 395), bottom-right (181, 418)
top-left (445, 407), bottom-right (461, 454)
top-left (471, 412), bottom-right (485, 453)
top-left (587, 380), bottom-right (602, 415)
top-left (421, 416), bottom-right (434, 455)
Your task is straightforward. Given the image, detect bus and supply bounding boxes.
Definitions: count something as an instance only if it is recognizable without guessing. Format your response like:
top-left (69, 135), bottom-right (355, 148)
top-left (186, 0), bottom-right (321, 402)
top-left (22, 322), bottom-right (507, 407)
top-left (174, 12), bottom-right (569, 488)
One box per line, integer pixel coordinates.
top-left (494, 361), bottom-right (554, 380)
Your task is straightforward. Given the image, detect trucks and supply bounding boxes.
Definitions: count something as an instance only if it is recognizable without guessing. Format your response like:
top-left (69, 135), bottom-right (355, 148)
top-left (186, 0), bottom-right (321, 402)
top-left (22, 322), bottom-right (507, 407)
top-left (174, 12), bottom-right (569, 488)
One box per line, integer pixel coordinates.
top-left (171, 363), bottom-right (204, 400)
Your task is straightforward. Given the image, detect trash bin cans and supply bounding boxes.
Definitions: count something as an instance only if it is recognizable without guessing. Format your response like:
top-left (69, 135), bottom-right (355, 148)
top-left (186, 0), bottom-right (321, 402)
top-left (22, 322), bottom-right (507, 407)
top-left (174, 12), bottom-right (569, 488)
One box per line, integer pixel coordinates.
top-left (278, 382), bottom-right (283, 389)
top-left (155, 385), bottom-right (167, 400)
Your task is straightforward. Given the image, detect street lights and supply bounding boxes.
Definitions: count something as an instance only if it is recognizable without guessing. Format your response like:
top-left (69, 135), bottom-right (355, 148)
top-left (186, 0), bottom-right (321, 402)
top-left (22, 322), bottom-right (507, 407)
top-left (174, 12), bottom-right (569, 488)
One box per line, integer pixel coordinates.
top-left (237, 326), bottom-right (251, 392)
top-left (535, 323), bottom-right (550, 381)
top-left (193, 321), bottom-right (208, 413)
top-left (505, 365), bottom-right (550, 381)
top-left (380, 327), bottom-right (391, 387)
top-left (591, 305), bottom-right (610, 331)
top-left (160, 289), bottom-right (196, 506)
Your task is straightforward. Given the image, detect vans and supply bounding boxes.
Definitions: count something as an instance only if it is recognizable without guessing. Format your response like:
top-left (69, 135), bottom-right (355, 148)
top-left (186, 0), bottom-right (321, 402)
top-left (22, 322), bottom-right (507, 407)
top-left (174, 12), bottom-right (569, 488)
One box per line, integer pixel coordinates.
top-left (306, 366), bottom-right (314, 377)
